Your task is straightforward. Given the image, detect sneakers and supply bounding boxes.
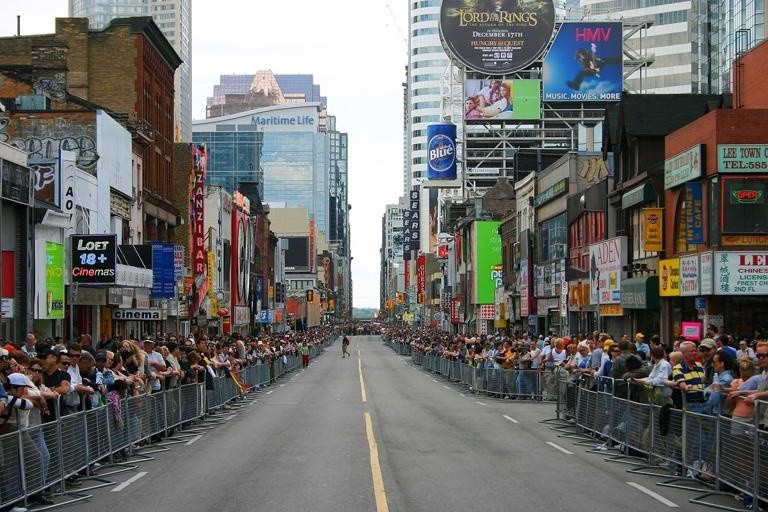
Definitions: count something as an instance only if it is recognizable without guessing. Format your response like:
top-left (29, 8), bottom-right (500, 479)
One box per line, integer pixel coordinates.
top-left (742, 492), bottom-right (756, 509)
top-left (719, 483), bottom-right (733, 492)
top-left (658, 460), bottom-right (672, 467)
top-left (488, 393), bottom-right (543, 401)
top-left (613, 443), bottom-right (622, 450)
top-left (699, 471), bottom-right (716, 482)
top-left (64, 426), bottom-right (178, 486)
top-left (568, 418), bottom-right (578, 424)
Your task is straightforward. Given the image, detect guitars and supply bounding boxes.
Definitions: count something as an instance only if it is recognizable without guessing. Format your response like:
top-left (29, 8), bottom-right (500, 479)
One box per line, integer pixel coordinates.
top-left (590, 43), bottom-right (602, 74)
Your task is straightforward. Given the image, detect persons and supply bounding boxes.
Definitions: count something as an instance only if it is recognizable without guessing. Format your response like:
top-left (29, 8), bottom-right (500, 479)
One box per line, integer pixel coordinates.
top-left (465, 79), bottom-right (513, 119)
top-left (566, 45), bottom-right (609, 91)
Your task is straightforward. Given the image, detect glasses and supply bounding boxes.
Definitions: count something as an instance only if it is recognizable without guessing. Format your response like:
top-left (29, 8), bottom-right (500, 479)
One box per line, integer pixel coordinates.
top-left (756, 352), bottom-right (768, 359)
top-left (31, 368), bottom-right (41, 373)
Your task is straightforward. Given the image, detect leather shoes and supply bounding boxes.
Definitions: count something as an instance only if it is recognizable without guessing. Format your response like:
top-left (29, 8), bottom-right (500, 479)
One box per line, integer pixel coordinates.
top-left (8, 506), bottom-right (28, 512)
top-left (51, 486), bottom-right (64, 494)
top-left (30, 495), bottom-right (54, 505)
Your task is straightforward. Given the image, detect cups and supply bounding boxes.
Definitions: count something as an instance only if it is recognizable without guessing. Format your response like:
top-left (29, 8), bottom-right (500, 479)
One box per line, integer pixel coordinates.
top-left (427, 124), bottom-right (457, 181)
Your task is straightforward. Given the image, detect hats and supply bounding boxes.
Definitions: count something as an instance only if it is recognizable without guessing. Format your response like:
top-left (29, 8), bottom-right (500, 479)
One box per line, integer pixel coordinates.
top-left (36, 332), bottom-right (263, 362)
top-left (700, 337), bottom-right (718, 350)
top-left (6, 372), bottom-right (34, 388)
top-left (486, 332), bottom-right (682, 356)
top-left (0, 345), bottom-right (17, 357)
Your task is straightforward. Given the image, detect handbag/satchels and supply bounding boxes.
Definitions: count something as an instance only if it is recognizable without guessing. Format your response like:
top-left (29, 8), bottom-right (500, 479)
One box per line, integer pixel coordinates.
top-left (0, 415), bottom-right (9, 433)
top-left (63, 391), bottom-right (81, 408)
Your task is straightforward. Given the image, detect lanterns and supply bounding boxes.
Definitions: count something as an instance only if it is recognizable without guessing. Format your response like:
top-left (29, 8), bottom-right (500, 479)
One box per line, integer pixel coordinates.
top-left (218, 309), bottom-right (225, 317)
top-left (223, 308), bottom-right (229, 315)
